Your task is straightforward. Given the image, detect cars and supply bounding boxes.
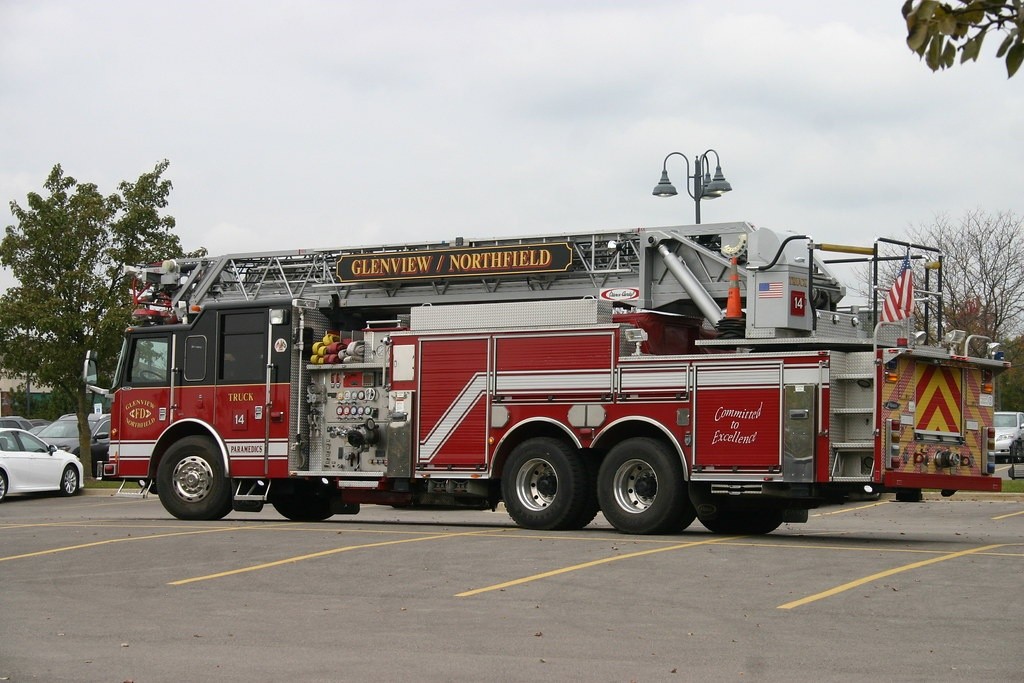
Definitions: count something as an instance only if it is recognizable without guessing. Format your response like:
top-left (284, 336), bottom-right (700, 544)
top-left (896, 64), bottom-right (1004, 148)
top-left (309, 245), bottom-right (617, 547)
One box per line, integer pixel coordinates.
top-left (991, 412), bottom-right (1024, 463)
top-left (0, 429), bottom-right (83, 501)
top-left (23, 419), bottom-right (51, 444)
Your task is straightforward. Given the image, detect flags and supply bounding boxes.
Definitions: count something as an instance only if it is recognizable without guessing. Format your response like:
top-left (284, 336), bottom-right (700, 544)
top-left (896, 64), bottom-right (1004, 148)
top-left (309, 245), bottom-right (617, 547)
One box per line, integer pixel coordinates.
top-left (880, 245), bottom-right (915, 328)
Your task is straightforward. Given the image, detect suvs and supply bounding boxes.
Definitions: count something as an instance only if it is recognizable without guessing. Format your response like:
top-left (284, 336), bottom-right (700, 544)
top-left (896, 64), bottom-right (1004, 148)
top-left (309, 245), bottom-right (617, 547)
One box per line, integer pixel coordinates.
top-left (0, 415), bottom-right (34, 436)
top-left (36, 412), bottom-right (110, 471)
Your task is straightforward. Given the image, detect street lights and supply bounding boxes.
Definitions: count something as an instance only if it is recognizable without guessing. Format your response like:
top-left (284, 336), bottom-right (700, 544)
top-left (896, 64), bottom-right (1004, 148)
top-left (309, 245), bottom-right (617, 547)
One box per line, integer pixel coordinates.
top-left (653, 150), bottom-right (731, 224)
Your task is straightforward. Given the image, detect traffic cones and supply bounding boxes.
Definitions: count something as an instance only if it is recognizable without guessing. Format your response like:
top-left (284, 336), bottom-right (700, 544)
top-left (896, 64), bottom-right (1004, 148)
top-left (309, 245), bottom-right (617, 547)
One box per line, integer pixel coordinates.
top-left (718, 256), bottom-right (745, 319)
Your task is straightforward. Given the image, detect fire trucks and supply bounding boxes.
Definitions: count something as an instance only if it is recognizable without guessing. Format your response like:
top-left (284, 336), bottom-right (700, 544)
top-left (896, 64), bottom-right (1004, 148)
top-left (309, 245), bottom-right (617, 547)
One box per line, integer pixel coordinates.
top-left (80, 222), bottom-right (1011, 535)
